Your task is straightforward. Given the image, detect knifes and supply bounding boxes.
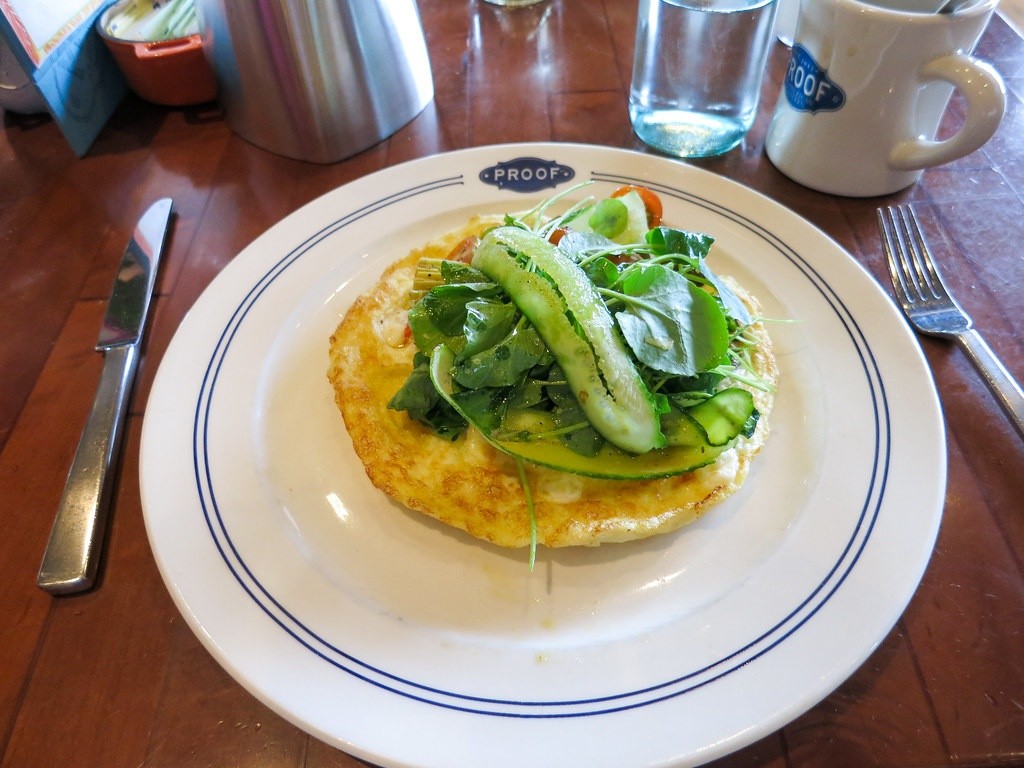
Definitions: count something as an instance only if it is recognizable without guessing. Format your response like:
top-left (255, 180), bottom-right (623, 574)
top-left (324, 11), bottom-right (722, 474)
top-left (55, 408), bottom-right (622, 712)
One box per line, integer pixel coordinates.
top-left (38, 198), bottom-right (173, 595)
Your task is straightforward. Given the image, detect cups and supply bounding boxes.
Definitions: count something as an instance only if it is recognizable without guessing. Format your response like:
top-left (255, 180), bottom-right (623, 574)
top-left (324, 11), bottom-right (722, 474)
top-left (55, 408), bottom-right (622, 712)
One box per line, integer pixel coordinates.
top-left (765, 0), bottom-right (1005, 199)
top-left (628, 1), bottom-right (778, 158)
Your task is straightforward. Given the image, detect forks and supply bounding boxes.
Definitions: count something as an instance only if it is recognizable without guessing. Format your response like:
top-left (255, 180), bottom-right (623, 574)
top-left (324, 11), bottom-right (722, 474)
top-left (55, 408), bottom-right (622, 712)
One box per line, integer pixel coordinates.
top-left (874, 204), bottom-right (1024, 441)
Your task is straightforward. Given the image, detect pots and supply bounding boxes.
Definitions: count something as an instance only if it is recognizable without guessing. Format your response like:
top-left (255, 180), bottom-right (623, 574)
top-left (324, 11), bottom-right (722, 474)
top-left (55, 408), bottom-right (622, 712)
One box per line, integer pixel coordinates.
top-left (96, 0), bottom-right (224, 107)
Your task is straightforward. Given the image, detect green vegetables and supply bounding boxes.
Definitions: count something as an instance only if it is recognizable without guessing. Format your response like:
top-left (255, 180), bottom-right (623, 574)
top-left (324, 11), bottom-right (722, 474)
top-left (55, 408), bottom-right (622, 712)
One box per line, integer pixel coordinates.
top-left (387, 181), bottom-right (800, 573)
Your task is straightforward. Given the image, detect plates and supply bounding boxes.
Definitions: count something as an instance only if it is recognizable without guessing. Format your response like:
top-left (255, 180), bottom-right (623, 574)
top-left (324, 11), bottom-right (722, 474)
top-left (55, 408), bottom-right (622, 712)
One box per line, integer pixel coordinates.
top-left (140, 143), bottom-right (950, 766)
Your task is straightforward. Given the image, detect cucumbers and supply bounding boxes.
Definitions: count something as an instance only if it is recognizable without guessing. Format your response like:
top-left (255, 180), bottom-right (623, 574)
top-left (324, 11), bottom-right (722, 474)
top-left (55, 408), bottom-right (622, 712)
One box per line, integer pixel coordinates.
top-left (472, 227), bottom-right (661, 455)
